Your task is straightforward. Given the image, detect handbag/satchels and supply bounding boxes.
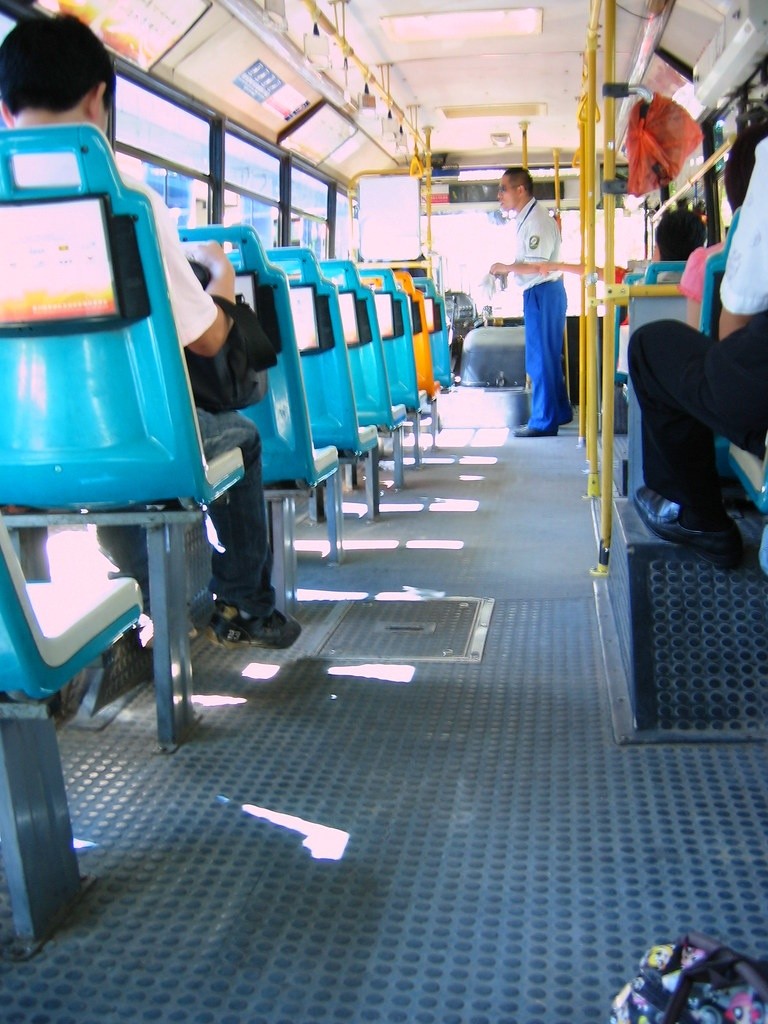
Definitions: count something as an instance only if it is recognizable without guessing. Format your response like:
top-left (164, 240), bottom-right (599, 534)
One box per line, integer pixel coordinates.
top-left (612, 933), bottom-right (766, 1022)
top-left (184, 260), bottom-right (282, 415)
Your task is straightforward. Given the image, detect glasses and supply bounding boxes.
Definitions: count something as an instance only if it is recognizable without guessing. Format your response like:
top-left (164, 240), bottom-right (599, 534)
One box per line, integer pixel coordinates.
top-left (496, 184), bottom-right (528, 193)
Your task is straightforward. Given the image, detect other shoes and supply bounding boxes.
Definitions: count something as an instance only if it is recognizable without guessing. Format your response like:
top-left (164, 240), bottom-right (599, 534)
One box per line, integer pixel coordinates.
top-left (558, 414), bottom-right (572, 425)
top-left (513, 425), bottom-right (557, 437)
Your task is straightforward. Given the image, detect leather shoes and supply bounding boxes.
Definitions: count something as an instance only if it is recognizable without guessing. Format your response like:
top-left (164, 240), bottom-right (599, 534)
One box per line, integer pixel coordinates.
top-left (632, 486), bottom-right (745, 566)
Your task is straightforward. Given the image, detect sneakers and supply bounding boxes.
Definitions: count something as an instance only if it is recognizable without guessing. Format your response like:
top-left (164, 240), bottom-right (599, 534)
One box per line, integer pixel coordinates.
top-left (204, 599), bottom-right (302, 650)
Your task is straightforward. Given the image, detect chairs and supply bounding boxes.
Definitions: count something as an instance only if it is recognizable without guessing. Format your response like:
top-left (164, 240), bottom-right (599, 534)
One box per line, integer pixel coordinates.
top-left (361, 270), bottom-right (442, 405)
top-left (0, 516), bottom-right (143, 703)
top-left (274, 259), bottom-right (408, 432)
top-left (614, 272), bottom-right (646, 388)
top-left (397, 277), bottom-right (455, 394)
top-left (226, 247), bottom-right (378, 464)
top-left (179, 224), bottom-right (340, 502)
top-left (0, 124), bottom-right (247, 511)
top-left (324, 268), bottom-right (428, 415)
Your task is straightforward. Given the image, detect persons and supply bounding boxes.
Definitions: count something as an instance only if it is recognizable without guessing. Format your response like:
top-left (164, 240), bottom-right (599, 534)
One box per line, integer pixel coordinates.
top-left (489, 166), bottom-right (574, 438)
top-left (540, 208), bottom-right (707, 325)
top-left (627, 132), bottom-right (768, 573)
top-left (677, 123), bottom-right (768, 332)
top-left (0, 14), bottom-right (305, 652)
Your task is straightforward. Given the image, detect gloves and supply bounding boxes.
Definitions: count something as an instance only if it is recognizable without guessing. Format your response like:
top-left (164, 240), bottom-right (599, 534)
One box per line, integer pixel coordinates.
top-left (477, 273), bottom-right (497, 300)
